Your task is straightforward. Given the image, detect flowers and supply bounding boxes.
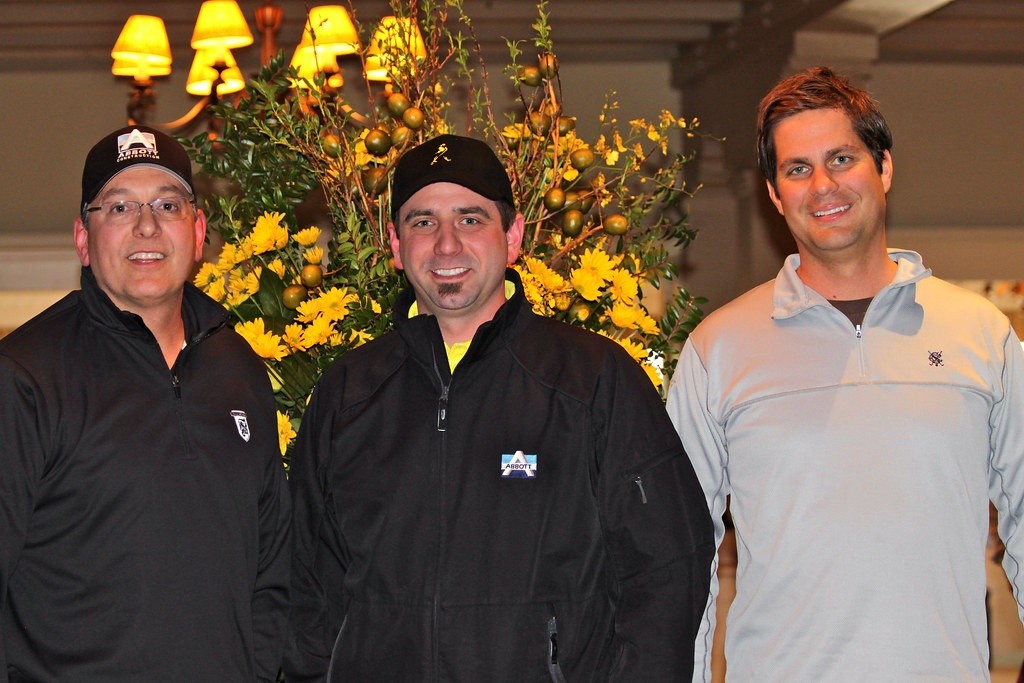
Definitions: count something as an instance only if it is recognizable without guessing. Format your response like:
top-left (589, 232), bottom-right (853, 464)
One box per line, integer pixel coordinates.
top-left (190, 0), bottom-right (728, 481)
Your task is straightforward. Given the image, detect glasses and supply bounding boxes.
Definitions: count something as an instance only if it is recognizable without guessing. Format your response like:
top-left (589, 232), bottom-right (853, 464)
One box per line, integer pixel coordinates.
top-left (84, 196), bottom-right (195, 226)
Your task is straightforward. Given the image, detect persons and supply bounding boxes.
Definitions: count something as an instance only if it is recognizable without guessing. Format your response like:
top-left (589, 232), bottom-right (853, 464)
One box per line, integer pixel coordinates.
top-left (663, 67), bottom-right (1024, 681)
top-left (288, 135), bottom-right (716, 683)
top-left (1, 124), bottom-right (291, 682)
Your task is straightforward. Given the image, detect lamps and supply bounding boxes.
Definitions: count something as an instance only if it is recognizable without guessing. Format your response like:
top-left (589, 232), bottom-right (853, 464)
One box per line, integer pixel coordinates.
top-left (186, 49), bottom-right (245, 96)
top-left (110, 14), bottom-right (172, 64)
top-left (367, 16), bottom-right (426, 64)
top-left (300, 5), bottom-right (360, 55)
top-left (362, 60), bottom-right (417, 82)
top-left (191, 1), bottom-right (254, 51)
top-left (110, 59), bottom-right (172, 79)
top-left (286, 44), bottom-right (345, 89)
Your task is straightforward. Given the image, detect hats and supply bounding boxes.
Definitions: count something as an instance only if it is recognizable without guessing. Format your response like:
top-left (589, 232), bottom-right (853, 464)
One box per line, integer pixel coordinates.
top-left (80, 126), bottom-right (198, 209)
top-left (392, 135), bottom-right (515, 219)
